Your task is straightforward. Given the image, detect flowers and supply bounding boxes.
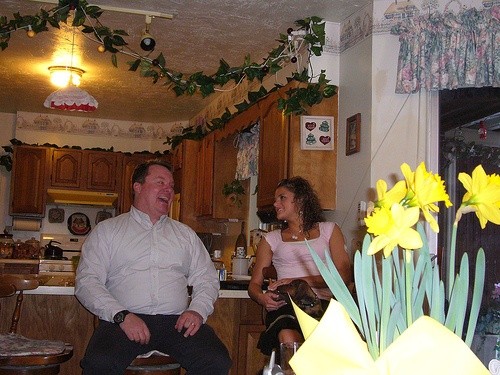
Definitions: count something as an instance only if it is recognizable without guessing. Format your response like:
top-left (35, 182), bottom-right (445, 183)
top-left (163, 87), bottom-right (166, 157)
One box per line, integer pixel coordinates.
top-left (471, 283), bottom-right (500, 335)
top-left (287, 161), bottom-right (500, 375)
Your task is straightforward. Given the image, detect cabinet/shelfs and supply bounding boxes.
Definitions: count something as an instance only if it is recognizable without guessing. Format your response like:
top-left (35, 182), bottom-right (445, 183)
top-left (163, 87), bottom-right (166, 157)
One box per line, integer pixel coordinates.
top-left (9, 80), bottom-right (337, 218)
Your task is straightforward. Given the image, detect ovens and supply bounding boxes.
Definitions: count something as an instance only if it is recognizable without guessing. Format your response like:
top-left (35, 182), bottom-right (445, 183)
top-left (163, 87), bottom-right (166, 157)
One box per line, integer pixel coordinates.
top-left (38, 263), bottom-right (75, 287)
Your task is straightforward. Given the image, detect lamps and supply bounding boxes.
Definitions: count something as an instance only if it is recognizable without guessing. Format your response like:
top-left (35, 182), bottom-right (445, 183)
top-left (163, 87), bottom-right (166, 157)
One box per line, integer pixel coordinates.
top-left (139, 15), bottom-right (156, 52)
top-left (48, 66), bottom-right (85, 88)
top-left (43, 12), bottom-right (99, 113)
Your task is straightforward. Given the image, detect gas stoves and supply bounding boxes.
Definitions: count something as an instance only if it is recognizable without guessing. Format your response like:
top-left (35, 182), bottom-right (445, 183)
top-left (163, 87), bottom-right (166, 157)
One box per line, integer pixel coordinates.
top-left (39, 233), bottom-right (88, 264)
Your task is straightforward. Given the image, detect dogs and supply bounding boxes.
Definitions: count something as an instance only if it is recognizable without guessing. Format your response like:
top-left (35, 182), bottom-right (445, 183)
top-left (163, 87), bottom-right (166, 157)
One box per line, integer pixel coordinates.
top-left (261, 278), bottom-right (326, 316)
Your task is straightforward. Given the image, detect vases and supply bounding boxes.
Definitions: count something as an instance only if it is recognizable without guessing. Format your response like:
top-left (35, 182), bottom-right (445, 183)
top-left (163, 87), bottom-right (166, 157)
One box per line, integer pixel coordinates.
top-left (472, 334), bottom-right (500, 369)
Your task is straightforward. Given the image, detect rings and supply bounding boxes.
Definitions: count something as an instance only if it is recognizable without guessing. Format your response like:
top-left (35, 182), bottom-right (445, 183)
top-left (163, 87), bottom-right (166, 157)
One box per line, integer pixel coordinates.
top-left (191, 323), bottom-right (196, 327)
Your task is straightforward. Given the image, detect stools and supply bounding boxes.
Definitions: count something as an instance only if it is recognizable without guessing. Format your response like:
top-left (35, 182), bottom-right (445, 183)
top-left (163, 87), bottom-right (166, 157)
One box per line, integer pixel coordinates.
top-left (0, 271), bottom-right (74, 375)
top-left (94, 314), bottom-right (182, 375)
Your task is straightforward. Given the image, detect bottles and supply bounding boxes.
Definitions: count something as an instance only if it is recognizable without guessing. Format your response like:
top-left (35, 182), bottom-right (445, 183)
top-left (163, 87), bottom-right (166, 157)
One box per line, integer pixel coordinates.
top-left (232, 258), bottom-right (249, 275)
top-left (0, 233), bottom-right (39, 260)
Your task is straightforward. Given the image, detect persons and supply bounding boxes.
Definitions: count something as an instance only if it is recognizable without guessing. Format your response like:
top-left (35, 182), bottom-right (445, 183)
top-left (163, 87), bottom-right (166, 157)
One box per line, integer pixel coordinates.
top-left (248, 176), bottom-right (351, 375)
top-left (74, 161), bottom-right (233, 375)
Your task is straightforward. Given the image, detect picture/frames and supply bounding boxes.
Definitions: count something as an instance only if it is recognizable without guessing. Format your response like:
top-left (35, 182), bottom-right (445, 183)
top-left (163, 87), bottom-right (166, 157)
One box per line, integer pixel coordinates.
top-left (346, 113), bottom-right (360, 156)
top-left (300, 116), bottom-right (335, 151)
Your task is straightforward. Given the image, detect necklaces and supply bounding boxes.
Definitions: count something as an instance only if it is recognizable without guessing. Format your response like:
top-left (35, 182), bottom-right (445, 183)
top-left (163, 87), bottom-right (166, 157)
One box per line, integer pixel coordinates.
top-left (286, 228), bottom-right (302, 240)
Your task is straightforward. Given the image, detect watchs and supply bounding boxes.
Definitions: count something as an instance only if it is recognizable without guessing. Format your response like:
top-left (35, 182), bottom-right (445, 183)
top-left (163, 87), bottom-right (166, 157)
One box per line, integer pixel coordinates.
top-left (113, 310), bottom-right (131, 326)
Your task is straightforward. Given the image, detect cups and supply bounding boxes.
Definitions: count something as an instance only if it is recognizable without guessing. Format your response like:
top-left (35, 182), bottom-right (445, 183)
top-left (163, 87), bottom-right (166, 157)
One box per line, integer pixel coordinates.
top-left (213, 250), bottom-right (221, 259)
top-left (216, 269), bottom-right (227, 281)
top-left (280, 342), bottom-right (302, 375)
top-left (236, 249), bottom-right (246, 258)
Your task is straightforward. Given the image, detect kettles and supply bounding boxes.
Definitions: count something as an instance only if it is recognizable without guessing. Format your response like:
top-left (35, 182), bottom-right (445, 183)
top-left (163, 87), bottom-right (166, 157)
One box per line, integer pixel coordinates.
top-left (44, 239), bottom-right (63, 259)
